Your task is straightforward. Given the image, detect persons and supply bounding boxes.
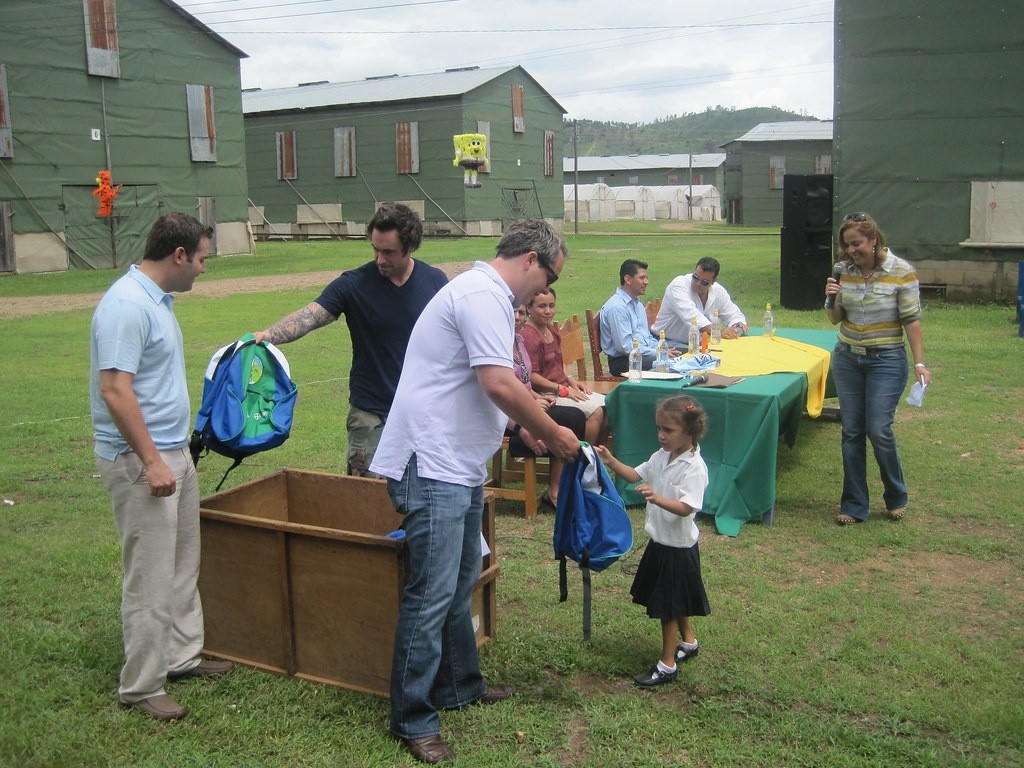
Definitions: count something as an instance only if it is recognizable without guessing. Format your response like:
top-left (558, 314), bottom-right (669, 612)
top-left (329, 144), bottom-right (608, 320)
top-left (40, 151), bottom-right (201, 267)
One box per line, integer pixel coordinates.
top-left (369, 218), bottom-right (580, 764)
top-left (592, 394), bottom-right (712, 687)
top-left (251, 203), bottom-right (449, 477)
top-left (650, 257), bottom-right (750, 352)
top-left (503, 287), bottom-right (612, 515)
top-left (90, 211), bottom-right (234, 721)
top-left (600, 259), bottom-right (682, 377)
top-left (824, 212), bottom-right (932, 524)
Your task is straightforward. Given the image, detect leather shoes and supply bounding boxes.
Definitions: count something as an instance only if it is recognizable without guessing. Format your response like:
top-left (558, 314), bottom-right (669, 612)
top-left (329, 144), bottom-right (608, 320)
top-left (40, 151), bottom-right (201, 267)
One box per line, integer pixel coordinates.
top-left (127, 694), bottom-right (186, 722)
top-left (634, 664), bottom-right (677, 688)
top-left (168, 661), bottom-right (234, 677)
top-left (473, 685), bottom-right (515, 703)
top-left (405, 734), bottom-right (452, 763)
top-left (674, 645), bottom-right (698, 663)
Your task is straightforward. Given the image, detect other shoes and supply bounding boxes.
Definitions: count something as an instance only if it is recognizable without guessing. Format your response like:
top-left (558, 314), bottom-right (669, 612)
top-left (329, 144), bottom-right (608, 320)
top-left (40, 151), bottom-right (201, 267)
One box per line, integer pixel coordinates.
top-left (888, 506), bottom-right (906, 519)
top-left (836, 513), bottom-right (858, 523)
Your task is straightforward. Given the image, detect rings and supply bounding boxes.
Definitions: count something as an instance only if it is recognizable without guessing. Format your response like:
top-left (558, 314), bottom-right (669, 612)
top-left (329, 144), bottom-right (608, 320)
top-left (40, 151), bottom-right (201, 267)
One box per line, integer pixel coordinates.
top-left (928, 379), bottom-right (932, 383)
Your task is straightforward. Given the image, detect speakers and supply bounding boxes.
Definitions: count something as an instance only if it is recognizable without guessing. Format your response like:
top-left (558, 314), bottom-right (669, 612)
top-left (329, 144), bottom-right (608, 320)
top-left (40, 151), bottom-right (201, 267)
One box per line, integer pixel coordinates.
top-left (780, 173), bottom-right (834, 311)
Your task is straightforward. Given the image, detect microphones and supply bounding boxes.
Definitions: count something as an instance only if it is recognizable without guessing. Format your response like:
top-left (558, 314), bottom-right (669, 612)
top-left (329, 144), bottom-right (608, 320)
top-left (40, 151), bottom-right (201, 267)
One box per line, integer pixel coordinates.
top-left (826, 262), bottom-right (844, 309)
top-left (682, 374), bottom-right (709, 387)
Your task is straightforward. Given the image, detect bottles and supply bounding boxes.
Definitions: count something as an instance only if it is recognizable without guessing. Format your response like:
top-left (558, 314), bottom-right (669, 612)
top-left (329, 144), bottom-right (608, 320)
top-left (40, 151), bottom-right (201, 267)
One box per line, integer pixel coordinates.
top-left (629, 339), bottom-right (642, 384)
top-left (656, 330), bottom-right (669, 362)
top-left (763, 303), bottom-right (773, 337)
top-left (711, 309), bottom-right (720, 345)
top-left (688, 318), bottom-right (699, 354)
top-left (701, 332), bottom-right (709, 354)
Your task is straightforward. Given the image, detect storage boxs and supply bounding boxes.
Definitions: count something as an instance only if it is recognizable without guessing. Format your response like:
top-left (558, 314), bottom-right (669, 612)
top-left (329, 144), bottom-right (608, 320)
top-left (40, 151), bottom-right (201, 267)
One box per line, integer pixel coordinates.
top-left (196, 467), bottom-right (500, 697)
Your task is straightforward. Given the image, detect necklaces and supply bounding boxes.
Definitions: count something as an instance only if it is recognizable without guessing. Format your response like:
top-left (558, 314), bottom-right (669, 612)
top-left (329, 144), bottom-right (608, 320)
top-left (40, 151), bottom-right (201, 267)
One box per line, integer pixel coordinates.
top-left (529, 318), bottom-right (549, 337)
top-left (863, 271), bottom-right (874, 279)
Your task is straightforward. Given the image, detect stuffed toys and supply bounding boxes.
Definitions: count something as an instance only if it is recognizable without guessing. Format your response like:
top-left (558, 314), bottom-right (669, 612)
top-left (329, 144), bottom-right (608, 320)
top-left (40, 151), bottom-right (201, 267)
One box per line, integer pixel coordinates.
top-left (92, 171), bottom-right (122, 216)
top-left (453, 133), bottom-right (488, 188)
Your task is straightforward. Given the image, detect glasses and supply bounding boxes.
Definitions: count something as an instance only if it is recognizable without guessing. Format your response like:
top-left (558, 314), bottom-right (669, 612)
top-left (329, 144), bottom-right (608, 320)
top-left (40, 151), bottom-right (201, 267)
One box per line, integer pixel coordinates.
top-left (538, 255), bottom-right (559, 286)
top-left (844, 213), bottom-right (866, 221)
top-left (692, 274), bottom-right (708, 285)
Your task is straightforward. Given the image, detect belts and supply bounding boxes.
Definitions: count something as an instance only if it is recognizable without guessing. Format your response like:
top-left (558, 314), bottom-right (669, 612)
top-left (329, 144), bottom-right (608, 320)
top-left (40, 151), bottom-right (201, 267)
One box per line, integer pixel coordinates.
top-left (847, 344), bottom-right (893, 353)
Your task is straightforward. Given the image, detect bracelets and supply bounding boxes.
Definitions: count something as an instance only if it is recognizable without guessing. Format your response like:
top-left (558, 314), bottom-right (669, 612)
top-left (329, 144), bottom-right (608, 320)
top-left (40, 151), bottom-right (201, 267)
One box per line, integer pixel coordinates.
top-left (559, 385), bottom-right (569, 397)
top-left (557, 382), bottom-right (560, 394)
top-left (514, 423), bottom-right (521, 437)
top-left (566, 374), bottom-right (573, 382)
top-left (913, 363), bottom-right (925, 371)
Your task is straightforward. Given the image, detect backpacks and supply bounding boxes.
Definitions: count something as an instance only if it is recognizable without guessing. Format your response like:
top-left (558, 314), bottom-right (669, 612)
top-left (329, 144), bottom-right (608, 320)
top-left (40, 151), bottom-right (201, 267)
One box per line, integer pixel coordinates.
top-left (190, 333), bottom-right (297, 493)
top-left (547, 441), bottom-right (633, 602)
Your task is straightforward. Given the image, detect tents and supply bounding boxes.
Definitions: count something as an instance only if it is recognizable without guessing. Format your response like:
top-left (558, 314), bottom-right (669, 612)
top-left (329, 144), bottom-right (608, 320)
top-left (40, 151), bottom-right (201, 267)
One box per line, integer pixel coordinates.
top-left (563, 183), bottom-right (721, 222)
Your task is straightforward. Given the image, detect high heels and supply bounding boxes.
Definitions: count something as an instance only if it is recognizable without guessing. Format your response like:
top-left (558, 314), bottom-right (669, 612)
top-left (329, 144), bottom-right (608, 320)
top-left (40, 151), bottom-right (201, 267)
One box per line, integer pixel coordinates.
top-left (542, 492), bottom-right (558, 513)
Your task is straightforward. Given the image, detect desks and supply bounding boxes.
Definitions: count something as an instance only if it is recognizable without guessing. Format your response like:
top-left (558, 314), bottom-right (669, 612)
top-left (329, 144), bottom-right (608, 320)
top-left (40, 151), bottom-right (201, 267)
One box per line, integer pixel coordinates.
top-left (604, 326), bottom-right (837, 539)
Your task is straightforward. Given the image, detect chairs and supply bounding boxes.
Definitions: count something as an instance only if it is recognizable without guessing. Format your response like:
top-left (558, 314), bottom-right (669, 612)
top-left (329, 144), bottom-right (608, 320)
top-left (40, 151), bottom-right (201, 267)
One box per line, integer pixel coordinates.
top-left (505, 450), bottom-right (550, 473)
top-left (644, 298), bottom-right (661, 329)
top-left (483, 437), bottom-right (550, 520)
top-left (554, 314), bottom-right (622, 449)
top-left (585, 309), bottom-right (630, 382)
